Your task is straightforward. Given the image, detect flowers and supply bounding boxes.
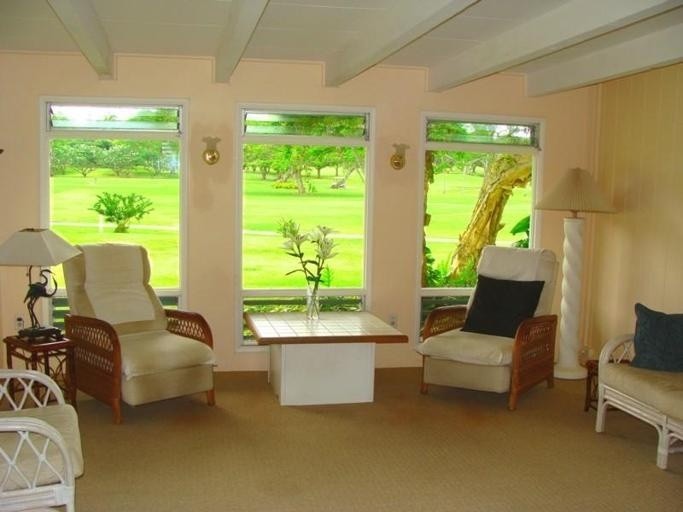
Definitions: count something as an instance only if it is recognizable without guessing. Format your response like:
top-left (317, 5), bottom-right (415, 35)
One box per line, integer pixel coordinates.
top-left (274, 213), bottom-right (340, 315)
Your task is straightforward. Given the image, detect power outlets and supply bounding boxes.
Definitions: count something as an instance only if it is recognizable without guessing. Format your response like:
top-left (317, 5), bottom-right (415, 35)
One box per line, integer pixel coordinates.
top-left (14, 314), bottom-right (24, 331)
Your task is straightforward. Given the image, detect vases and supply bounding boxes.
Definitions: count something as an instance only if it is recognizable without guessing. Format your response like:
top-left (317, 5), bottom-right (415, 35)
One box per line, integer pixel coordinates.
top-left (303, 284), bottom-right (321, 320)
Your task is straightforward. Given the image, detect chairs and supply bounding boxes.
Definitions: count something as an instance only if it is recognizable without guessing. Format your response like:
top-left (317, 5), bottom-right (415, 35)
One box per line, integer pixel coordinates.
top-left (59, 242), bottom-right (219, 427)
top-left (0, 366), bottom-right (85, 512)
top-left (416, 241), bottom-right (565, 413)
top-left (593, 331), bottom-right (681, 475)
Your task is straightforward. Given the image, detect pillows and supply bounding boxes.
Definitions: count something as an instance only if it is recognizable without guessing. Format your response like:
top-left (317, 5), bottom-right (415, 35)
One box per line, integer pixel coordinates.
top-left (628, 298), bottom-right (682, 375)
top-left (459, 271), bottom-right (545, 340)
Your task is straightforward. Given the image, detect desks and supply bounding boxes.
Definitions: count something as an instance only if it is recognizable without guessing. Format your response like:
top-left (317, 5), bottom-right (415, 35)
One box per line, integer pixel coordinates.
top-left (2, 334), bottom-right (80, 413)
top-left (242, 307), bottom-right (408, 408)
top-left (581, 354), bottom-right (626, 416)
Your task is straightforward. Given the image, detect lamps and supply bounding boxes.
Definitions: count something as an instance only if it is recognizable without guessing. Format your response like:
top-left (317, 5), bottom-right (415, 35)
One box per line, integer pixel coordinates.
top-left (0, 225), bottom-right (82, 343)
top-left (530, 162), bottom-right (624, 384)
